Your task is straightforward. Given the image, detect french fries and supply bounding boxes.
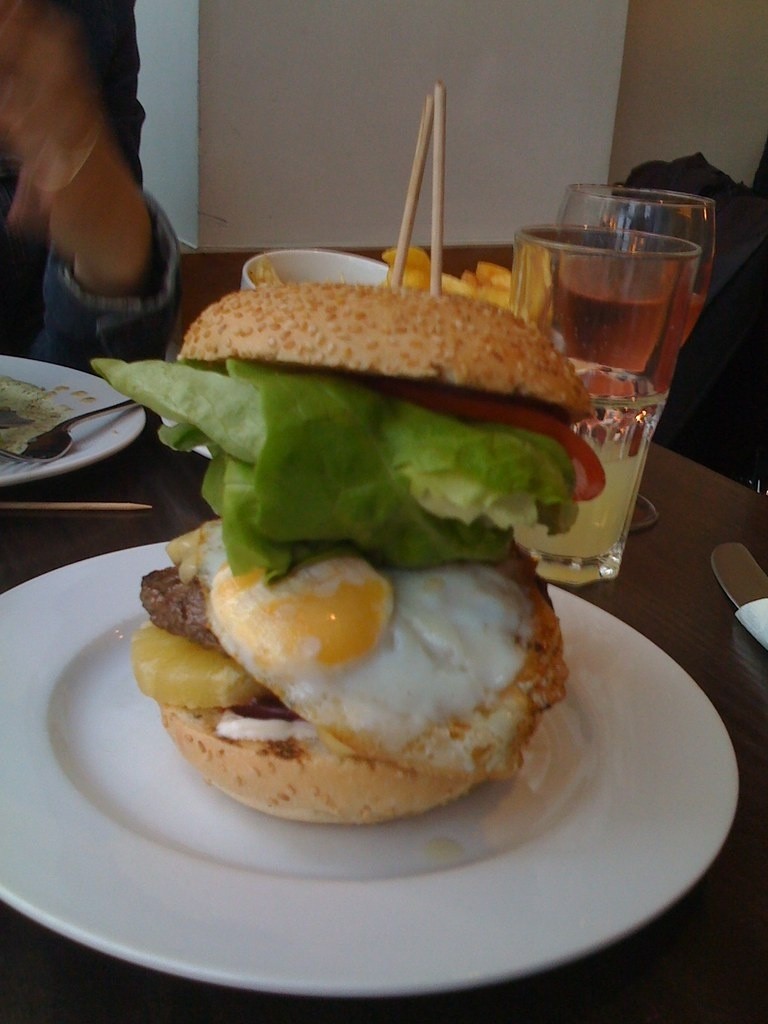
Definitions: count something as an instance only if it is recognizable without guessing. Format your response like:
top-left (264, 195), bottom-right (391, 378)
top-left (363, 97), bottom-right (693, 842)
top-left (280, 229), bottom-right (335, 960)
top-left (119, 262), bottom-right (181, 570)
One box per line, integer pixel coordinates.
top-left (383, 248), bottom-right (517, 310)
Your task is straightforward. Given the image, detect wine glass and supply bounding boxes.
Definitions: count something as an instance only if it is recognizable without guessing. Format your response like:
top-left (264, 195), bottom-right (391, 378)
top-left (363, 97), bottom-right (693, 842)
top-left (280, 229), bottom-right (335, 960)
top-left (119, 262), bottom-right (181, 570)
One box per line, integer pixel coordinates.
top-left (558, 185), bottom-right (716, 532)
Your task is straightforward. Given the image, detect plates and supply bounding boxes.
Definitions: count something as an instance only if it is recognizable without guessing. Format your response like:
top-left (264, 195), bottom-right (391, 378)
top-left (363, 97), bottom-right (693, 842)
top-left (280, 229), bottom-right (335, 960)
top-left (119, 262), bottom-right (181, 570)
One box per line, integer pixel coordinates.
top-left (161, 416), bottom-right (213, 459)
top-left (1, 542), bottom-right (739, 998)
top-left (1, 355), bottom-right (146, 485)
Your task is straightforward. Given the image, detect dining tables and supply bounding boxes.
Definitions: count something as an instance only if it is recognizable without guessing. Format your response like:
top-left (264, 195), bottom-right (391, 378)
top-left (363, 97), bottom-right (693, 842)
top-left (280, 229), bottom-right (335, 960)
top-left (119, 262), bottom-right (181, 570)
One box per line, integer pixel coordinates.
top-left (0, 404), bottom-right (768, 1022)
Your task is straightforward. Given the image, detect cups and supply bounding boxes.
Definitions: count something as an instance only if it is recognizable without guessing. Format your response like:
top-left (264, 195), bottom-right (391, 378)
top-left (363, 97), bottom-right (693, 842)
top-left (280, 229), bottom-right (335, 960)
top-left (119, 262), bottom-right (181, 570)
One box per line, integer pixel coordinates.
top-left (241, 250), bottom-right (391, 288)
top-left (509, 224), bottom-right (700, 587)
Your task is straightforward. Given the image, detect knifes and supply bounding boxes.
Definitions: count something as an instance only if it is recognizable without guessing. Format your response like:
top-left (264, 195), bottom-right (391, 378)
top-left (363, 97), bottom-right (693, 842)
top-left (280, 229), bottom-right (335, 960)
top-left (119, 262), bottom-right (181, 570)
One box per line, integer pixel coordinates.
top-left (711, 541), bottom-right (768, 650)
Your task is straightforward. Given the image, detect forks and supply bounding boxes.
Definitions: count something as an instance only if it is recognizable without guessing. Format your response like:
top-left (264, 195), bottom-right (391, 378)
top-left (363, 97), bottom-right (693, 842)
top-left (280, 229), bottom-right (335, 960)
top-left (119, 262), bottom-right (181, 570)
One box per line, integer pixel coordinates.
top-left (0, 399), bottom-right (141, 463)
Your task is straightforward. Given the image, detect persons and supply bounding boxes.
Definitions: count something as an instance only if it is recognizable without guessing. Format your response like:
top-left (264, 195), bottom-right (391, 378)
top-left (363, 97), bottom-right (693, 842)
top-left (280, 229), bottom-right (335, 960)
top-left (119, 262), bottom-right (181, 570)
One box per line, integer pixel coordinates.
top-left (0, 0), bottom-right (182, 377)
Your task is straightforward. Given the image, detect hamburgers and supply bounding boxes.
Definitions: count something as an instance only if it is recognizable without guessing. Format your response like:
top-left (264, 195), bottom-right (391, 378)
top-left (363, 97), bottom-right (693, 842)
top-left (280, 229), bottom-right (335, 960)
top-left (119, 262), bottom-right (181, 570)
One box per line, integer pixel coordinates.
top-left (89, 284), bottom-right (607, 825)
